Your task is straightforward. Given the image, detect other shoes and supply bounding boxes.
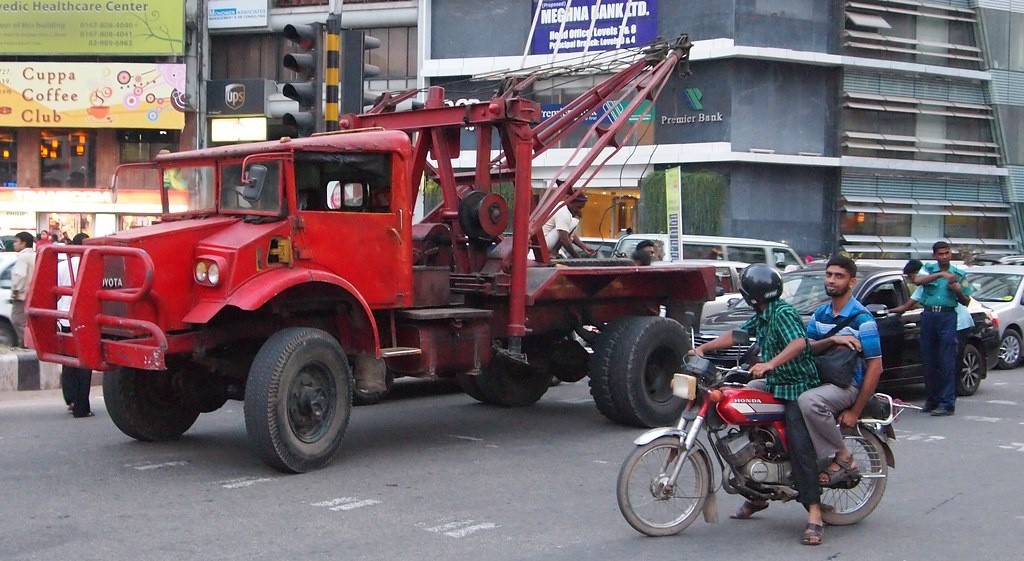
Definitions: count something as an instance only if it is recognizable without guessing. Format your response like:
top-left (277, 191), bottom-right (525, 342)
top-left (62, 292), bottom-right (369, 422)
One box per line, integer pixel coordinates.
top-left (932, 408), bottom-right (955, 416)
top-left (923, 403), bottom-right (936, 413)
top-left (68, 402), bottom-right (75, 410)
top-left (75, 411), bottom-right (95, 418)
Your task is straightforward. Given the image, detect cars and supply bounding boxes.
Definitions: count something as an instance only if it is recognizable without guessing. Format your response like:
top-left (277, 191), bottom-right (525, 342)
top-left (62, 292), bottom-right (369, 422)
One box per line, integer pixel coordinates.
top-left (0, 237), bottom-right (67, 345)
top-left (645, 254), bottom-right (1024, 397)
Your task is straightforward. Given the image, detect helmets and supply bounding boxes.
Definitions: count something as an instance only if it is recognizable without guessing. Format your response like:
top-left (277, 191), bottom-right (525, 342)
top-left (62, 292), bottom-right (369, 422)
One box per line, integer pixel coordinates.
top-left (739, 263), bottom-right (782, 308)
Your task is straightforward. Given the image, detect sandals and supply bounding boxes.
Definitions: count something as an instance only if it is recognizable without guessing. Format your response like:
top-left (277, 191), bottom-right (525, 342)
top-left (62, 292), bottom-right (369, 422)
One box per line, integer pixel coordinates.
top-left (818, 453), bottom-right (859, 486)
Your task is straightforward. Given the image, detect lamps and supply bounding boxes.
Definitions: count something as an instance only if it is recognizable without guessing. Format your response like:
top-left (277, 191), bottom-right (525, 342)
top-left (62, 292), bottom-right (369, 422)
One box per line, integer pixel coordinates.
top-left (4, 136), bottom-right (87, 159)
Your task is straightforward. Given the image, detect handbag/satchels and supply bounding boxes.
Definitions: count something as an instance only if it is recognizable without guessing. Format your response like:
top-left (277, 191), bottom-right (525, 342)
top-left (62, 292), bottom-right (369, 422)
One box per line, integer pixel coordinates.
top-left (814, 343), bottom-right (859, 390)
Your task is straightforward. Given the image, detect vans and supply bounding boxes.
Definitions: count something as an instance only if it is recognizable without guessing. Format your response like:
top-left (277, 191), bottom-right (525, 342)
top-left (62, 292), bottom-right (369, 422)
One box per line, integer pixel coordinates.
top-left (525, 234), bottom-right (803, 326)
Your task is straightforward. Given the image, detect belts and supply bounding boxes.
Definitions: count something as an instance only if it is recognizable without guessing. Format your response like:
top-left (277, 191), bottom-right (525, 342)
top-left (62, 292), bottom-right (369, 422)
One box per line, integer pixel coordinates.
top-left (924, 306), bottom-right (955, 314)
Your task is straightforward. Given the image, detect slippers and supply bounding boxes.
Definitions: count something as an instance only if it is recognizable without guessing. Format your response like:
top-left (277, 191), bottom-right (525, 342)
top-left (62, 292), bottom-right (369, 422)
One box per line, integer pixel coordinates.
top-left (731, 501), bottom-right (769, 519)
top-left (802, 522), bottom-right (823, 545)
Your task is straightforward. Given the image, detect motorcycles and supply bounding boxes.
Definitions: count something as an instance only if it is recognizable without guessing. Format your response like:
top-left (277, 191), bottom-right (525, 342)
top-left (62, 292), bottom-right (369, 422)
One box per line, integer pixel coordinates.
top-left (617, 311), bottom-right (923, 537)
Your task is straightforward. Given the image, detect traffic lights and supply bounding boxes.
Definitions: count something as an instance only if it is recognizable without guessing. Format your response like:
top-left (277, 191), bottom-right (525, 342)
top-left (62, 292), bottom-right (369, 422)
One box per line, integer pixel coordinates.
top-left (338, 30), bottom-right (381, 116)
top-left (283, 23), bottom-right (326, 137)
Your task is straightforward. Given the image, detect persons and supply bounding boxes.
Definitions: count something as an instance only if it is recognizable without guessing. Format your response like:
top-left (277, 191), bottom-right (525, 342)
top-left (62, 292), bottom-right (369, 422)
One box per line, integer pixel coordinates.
top-left (797, 257), bottom-right (884, 486)
top-left (914, 241), bottom-right (972, 417)
top-left (688, 263), bottom-right (825, 545)
top-left (542, 194), bottom-right (596, 259)
top-left (57, 233), bottom-right (95, 418)
top-left (631, 249), bottom-right (651, 265)
top-left (888, 259), bottom-right (976, 400)
top-left (636, 239), bottom-right (654, 259)
top-left (10, 232), bottom-right (36, 347)
top-left (35, 230), bottom-right (73, 247)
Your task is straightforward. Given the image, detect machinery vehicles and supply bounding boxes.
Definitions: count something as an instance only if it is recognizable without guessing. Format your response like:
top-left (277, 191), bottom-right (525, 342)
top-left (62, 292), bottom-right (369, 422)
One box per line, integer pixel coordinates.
top-left (22, 33), bottom-right (741, 475)
